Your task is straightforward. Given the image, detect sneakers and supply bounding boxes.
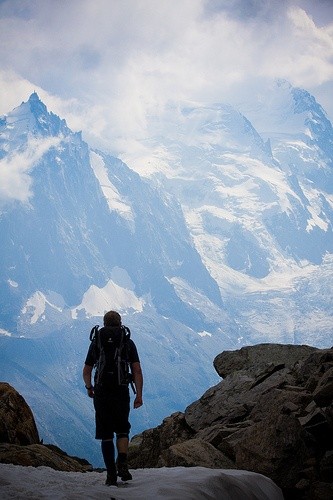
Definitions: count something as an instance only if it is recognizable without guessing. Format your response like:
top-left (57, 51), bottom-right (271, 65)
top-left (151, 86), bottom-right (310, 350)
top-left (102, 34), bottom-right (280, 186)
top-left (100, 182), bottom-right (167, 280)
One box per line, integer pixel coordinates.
top-left (118, 465), bottom-right (132, 481)
top-left (106, 473), bottom-right (117, 485)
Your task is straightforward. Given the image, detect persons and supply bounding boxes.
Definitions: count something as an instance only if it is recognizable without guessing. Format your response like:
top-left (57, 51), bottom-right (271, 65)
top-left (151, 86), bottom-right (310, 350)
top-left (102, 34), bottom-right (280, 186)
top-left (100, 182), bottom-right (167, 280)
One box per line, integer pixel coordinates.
top-left (83, 311), bottom-right (143, 487)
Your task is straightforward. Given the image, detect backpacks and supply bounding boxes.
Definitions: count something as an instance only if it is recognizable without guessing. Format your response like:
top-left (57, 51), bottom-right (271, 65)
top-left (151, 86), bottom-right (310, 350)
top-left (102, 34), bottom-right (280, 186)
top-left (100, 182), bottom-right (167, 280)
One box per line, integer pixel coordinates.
top-left (90, 325), bottom-right (132, 391)
